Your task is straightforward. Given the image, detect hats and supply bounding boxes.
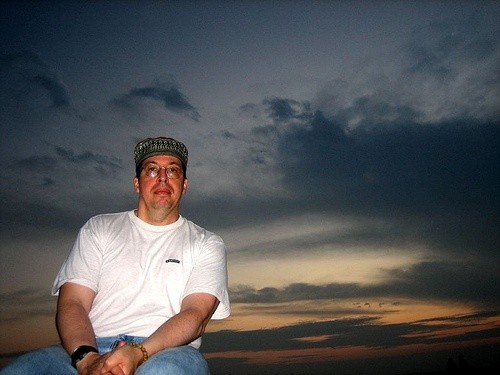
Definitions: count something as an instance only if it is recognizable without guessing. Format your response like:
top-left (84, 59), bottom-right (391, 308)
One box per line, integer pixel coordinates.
top-left (134, 136), bottom-right (188, 167)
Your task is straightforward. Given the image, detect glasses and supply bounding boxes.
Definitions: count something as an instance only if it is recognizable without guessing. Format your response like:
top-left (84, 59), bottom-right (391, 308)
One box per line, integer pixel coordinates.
top-left (142, 164), bottom-right (184, 179)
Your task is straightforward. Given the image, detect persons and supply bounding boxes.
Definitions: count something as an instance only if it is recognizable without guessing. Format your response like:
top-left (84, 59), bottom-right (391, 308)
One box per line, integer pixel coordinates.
top-left (1, 136), bottom-right (234, 375)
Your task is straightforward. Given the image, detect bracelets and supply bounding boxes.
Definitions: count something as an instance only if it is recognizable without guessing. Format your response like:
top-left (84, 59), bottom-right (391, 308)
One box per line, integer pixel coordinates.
top-left (127, 340), bottom-right (148, 364)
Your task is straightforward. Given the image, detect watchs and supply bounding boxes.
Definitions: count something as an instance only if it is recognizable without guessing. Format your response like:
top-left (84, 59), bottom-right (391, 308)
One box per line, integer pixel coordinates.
top-left (71, 345), bottom-right (100, 370)
